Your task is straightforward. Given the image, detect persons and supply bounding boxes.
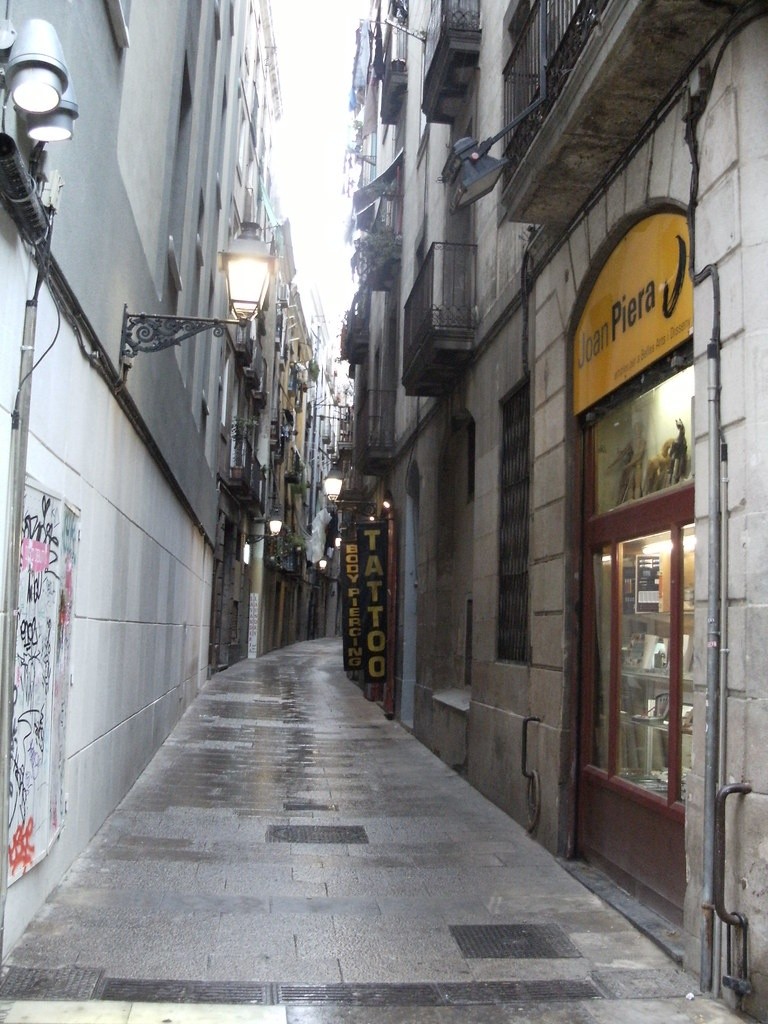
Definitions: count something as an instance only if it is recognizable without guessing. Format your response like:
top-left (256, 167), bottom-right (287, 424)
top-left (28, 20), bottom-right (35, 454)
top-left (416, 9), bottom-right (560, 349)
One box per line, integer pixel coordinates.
top-left (608, 422), bottom-right (646, 504)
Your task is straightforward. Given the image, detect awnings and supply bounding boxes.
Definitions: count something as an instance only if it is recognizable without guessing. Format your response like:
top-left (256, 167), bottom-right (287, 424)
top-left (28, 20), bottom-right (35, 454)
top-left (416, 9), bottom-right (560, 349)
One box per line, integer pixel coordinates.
top-left (261, 179), bottom-right (283, 250)
top-left (344, 146), bottom-right (404, 243)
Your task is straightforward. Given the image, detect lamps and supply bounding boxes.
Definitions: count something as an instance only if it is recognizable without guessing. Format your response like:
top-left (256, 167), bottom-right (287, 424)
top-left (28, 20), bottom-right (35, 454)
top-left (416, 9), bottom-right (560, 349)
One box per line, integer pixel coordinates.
top-left (117, 218), bottom-right (277, 385)
top-left (309, 557), bottom-right (330, 574)
top-left (325, 466), bottom-right (376, 509)
top-left (439, 1), bottom-right (546, 216)
top-left (245, 505), bottom-right (284, 543)
top-left (2, 17), bottom-right (80, 143)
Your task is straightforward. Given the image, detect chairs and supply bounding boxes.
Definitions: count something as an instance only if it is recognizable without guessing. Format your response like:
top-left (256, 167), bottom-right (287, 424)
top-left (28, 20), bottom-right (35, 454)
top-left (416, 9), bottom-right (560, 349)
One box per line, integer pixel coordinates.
top-left (633, 692), bottom-right (671, 779)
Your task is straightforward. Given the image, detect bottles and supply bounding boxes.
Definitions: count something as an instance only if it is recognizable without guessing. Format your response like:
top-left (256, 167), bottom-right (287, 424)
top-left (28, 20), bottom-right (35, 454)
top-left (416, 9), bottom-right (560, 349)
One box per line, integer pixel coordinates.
top-left (652, 636), bottom-right (668, 669)
